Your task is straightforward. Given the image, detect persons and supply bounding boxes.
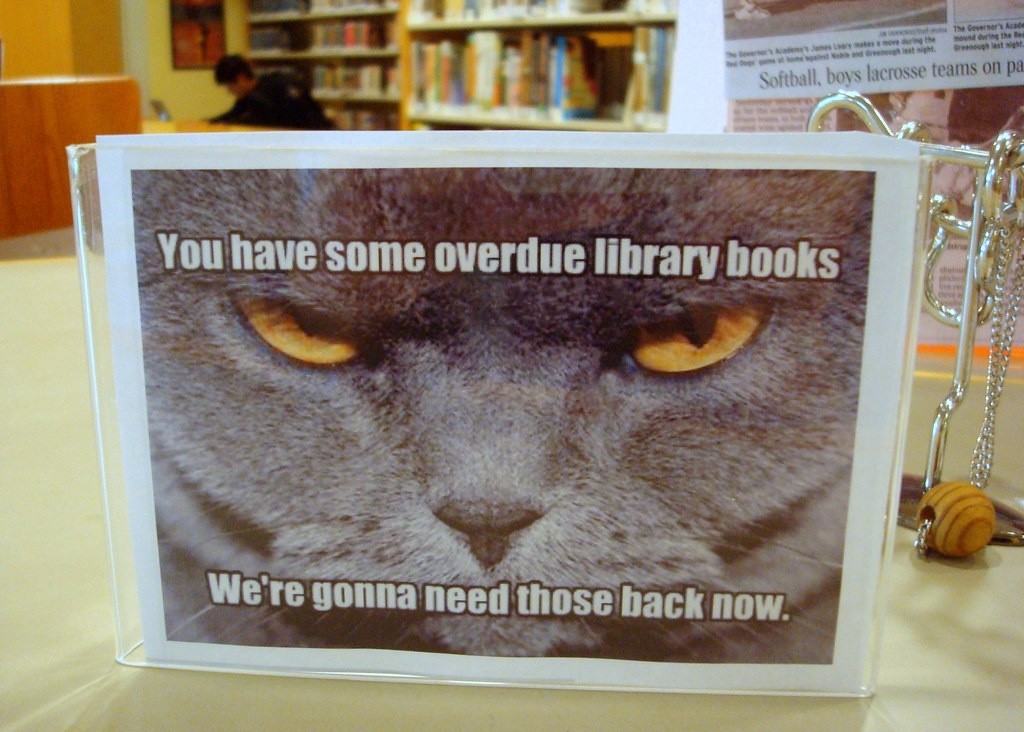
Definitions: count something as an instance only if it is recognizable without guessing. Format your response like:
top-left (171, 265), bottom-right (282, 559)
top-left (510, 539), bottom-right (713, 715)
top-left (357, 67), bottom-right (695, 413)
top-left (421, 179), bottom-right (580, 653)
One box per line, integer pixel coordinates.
top-left (186, 53), bottom-right (332, 130)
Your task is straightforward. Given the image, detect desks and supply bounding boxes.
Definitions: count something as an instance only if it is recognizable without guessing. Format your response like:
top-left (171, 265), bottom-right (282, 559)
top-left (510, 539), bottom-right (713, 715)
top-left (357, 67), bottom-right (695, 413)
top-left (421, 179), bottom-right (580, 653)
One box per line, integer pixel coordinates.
top-left (0, 75), bottom-right (142, 239)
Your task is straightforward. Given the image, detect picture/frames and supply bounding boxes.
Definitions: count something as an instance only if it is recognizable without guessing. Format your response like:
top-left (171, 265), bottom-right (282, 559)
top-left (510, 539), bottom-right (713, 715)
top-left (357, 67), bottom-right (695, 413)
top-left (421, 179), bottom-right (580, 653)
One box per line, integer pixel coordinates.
top-left (170, 0), bottom-right (226, 70)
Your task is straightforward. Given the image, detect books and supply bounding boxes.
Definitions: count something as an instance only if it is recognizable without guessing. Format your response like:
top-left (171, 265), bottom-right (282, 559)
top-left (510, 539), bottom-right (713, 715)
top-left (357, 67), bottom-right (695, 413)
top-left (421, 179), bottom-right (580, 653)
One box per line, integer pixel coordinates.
top-left (303, 0), bottom-right (676, 124)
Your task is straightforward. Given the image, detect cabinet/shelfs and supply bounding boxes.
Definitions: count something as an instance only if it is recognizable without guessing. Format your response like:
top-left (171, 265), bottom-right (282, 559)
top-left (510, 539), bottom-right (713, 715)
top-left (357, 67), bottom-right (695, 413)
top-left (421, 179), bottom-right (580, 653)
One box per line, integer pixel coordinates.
top-left (226, 0), bottom-right (679, 135)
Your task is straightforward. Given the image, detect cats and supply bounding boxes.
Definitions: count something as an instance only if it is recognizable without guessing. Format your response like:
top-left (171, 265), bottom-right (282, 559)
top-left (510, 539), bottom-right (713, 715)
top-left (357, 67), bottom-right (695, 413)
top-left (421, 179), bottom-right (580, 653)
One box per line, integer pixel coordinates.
top-left (131, 166), bottom-right (874, 664)
